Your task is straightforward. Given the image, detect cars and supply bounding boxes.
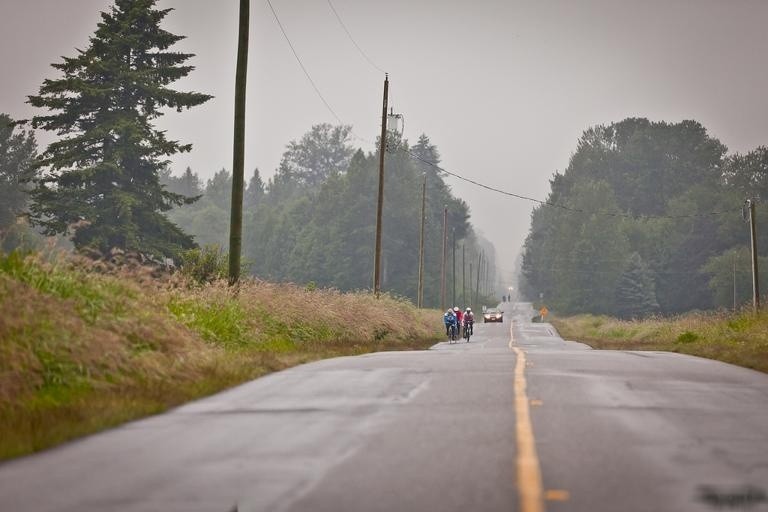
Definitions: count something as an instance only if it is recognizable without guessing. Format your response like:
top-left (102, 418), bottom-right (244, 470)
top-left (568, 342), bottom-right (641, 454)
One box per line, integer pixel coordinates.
top-left (481, 307), bottom-right (504, 323)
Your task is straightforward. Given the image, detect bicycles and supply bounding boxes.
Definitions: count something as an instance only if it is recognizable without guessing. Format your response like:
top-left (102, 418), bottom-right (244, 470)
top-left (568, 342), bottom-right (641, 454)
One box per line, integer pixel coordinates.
top-left (442, 320), bottom-right (475, 345)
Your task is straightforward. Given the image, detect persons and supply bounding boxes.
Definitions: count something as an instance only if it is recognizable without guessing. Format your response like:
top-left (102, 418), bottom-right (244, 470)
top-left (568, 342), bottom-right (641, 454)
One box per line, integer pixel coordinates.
top-left (462, 307), bottom-right (474, 338)
top-left (444, 308), bottom-right (458, 340)
top-left (453, 307), bottom-right (463, 335)
